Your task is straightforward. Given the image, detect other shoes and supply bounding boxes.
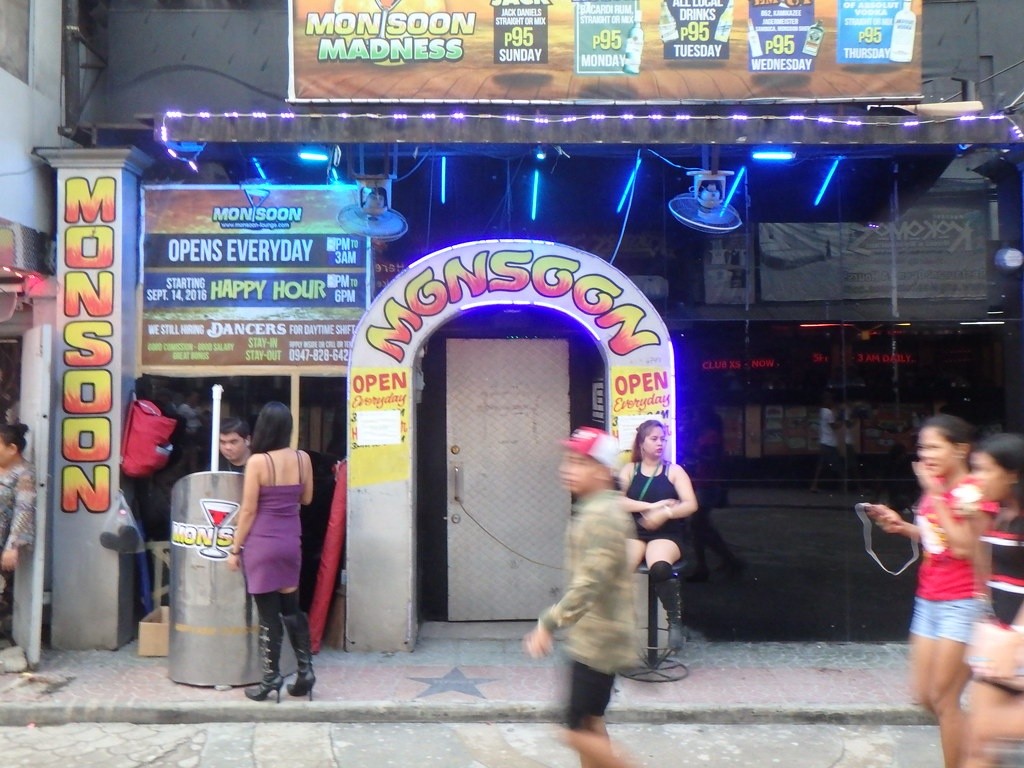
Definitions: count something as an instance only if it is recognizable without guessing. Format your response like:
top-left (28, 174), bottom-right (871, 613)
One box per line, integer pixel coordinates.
top-left (714, 558), bottom-right (743, 573)
top-left (682, 569), bottom-right (710, 582)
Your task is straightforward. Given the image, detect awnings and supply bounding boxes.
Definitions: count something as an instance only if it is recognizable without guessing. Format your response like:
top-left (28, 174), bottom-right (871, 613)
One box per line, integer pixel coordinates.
top-left (154, 104), bottom-right (1024, 161)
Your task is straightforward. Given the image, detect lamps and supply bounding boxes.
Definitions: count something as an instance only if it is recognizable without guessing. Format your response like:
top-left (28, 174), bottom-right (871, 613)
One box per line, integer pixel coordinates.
top-left (984, 179), bottom-right (1023, 272)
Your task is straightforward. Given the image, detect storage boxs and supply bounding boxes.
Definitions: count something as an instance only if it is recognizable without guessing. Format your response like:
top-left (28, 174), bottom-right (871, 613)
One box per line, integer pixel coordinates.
top-left (137, 607), bottom-right (171, 657)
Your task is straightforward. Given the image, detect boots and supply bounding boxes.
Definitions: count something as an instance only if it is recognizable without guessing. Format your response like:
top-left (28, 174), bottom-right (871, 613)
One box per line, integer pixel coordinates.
top-left (283, 611), bottom-right (315, 700)
top-left (652, 578), bottom-right (686, 652)
top-left (245, 621), bottom-right (284, 703)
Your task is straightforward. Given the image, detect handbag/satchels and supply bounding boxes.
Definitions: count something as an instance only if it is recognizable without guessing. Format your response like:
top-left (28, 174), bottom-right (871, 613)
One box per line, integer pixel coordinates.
top-left (120, 391), bottom-right (176, 478)
top-left (99, 489), bottom-right (146, 554)
top-left (966, 618), bottom-right (1024, 694)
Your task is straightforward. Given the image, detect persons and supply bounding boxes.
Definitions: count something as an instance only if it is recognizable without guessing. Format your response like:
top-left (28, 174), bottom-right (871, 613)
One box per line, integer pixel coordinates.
top-left (219, 402), bottom-right (316, 703)
top-left (619, 420), bottom-right (697, 651)
top-left (0, 424), bottom-right (36, 638)
top-left (523, 426), bottom-right (647, 768)
top-left (810, 393), bottom-right (853, 493)
top-left (867, 416), bottom-right (1024, 768)
top-left (155, 386), bottom-right (212, 471)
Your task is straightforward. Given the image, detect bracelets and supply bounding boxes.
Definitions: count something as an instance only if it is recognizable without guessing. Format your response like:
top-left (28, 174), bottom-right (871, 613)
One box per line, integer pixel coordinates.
top-left (929, 495), bottom-right (949, 503)
top-left (664, 506), bottom-right (674, 519)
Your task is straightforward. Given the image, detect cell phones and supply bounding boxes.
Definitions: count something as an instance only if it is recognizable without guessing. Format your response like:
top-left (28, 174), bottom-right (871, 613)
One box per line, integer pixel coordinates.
top-left (856, 502), bottom-right (904, 527)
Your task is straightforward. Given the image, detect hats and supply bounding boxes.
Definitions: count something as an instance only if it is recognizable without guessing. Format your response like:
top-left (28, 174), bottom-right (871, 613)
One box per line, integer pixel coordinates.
top-left (557, 426), bottom-right (625, 475)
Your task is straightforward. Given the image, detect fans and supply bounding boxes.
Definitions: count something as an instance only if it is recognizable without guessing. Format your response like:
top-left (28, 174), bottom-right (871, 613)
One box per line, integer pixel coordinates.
top-left (337, 143), bottom-right (408, 243)
top-left (668, 144), bottom-right (743, 235)
